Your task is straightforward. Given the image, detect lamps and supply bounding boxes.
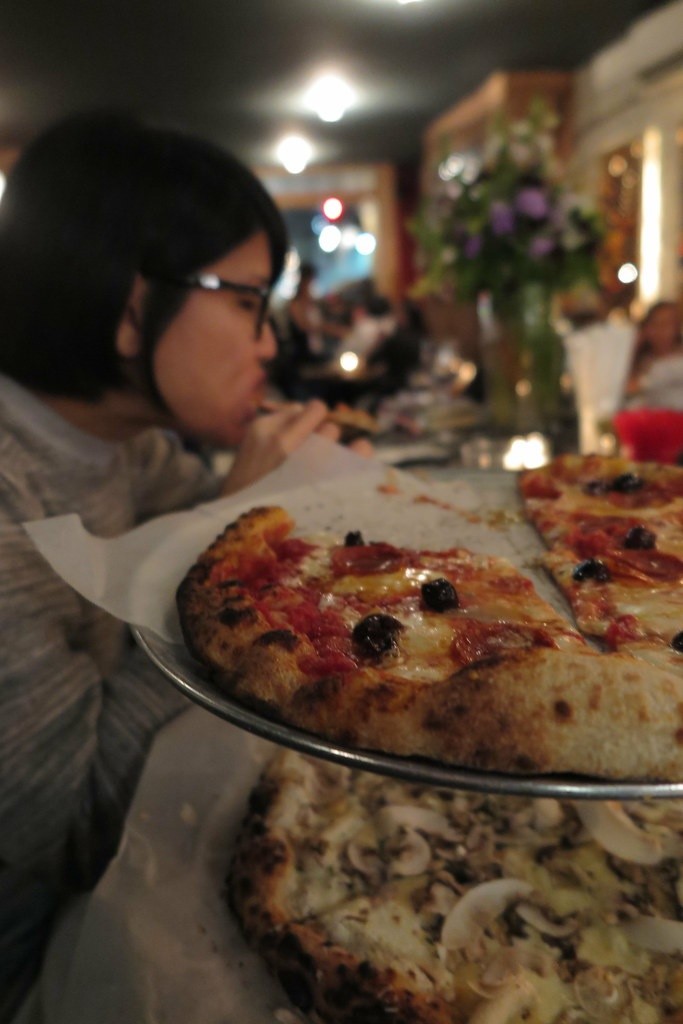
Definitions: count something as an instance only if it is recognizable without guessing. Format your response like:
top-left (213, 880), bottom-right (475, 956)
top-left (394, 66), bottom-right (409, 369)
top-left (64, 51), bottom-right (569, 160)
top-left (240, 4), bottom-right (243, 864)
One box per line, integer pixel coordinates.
top-left (277, 135), bottom-right (316, 174)
top-left (300, 67), bottom-right (363, 123)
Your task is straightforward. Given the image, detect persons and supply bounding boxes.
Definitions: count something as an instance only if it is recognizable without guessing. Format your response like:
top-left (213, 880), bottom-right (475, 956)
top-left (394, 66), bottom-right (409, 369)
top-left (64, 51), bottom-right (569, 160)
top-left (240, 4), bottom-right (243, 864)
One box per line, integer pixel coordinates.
top-left (0, 110), bottom-right (376, 1024)
top-left (287, 262), bottom-right (395, 396)
top-left (627, 302), bottom-right (683, 410)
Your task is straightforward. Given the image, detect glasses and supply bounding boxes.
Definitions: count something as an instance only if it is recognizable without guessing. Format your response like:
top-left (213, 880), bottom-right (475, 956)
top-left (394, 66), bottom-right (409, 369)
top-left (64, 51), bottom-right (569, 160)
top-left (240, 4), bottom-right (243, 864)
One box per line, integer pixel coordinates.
top-left (145, 272), bottom-right (271, 339)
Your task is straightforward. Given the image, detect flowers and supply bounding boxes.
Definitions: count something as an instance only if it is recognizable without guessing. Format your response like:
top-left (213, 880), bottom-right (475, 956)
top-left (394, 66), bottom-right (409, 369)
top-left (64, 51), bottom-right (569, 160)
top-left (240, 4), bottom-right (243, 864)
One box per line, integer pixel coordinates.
top-left (406, 95), bottom-right (605, 307)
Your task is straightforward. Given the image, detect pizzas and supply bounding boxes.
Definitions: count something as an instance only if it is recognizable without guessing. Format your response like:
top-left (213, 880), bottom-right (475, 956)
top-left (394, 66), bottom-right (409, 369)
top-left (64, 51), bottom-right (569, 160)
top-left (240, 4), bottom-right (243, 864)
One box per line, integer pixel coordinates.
top-left (176, 455), bottom-right (683, 783)
top-left (255, 397), bottom-right (380, 439)
top-left (227, 748), bottom-right (683, 1024)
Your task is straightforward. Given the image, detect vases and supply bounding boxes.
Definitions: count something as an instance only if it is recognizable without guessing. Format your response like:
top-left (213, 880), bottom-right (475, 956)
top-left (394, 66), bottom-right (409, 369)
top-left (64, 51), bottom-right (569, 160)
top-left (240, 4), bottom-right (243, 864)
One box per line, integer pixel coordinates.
top-left (472, 282), bottom-right (567, 435)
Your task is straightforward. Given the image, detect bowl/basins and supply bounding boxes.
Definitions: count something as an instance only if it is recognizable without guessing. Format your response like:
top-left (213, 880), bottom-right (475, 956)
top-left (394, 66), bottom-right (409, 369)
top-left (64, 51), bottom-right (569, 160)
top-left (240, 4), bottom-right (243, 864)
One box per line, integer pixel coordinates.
top-left (612, 408), bottom-right (683, 464)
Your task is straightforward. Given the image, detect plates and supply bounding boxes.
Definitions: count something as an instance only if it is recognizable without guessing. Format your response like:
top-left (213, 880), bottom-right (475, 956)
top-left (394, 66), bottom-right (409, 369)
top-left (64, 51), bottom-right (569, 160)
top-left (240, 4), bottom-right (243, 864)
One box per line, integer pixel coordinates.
top-left (129, 622), bottom-right (683, 800)
